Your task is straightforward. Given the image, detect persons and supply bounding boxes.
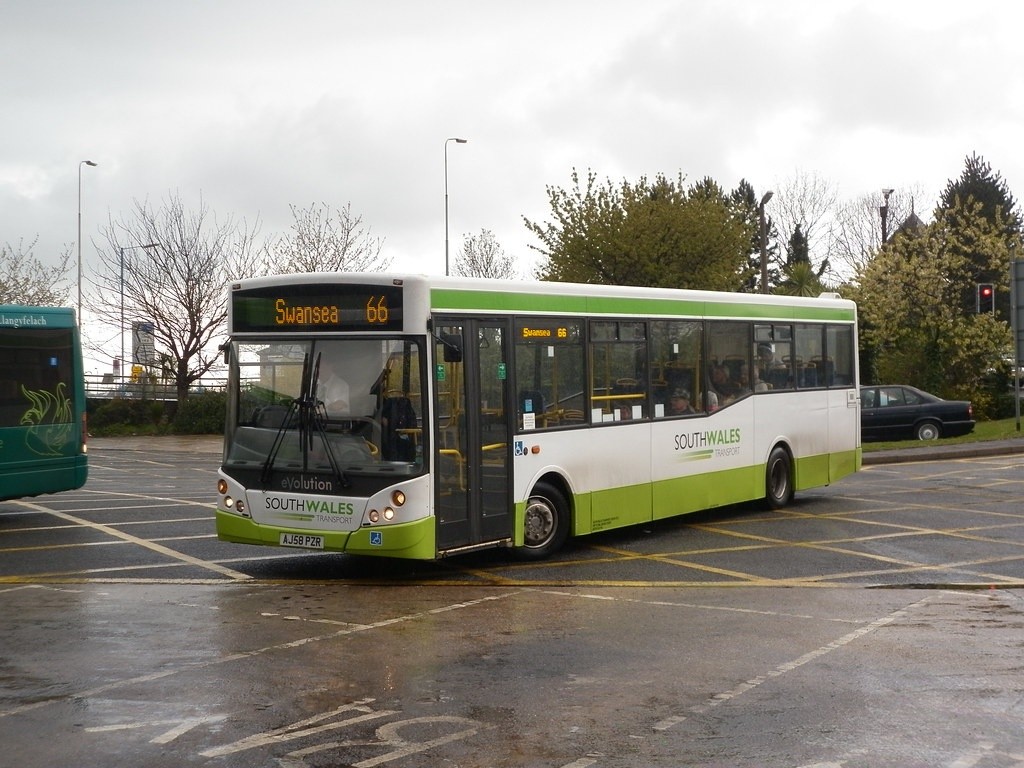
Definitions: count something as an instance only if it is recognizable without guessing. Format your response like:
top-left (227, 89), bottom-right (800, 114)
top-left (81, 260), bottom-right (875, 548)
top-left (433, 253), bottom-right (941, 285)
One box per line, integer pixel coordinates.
top-left (303, 350), bottom-right (351, 434)
top-left (615, 400), bottom-right (648, 420)
top-left (755, 342), bottom-right (787, 368)
top-left (738, 363), bottom-right (769, 393)
top-left (710, 363), bottom-right (744, 408)
top-left (666, 386), bottom-right (695, 416)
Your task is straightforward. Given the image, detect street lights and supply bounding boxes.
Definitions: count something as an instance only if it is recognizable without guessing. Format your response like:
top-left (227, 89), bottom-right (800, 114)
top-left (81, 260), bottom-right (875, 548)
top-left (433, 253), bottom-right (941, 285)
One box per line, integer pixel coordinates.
top-left (77, 158), bottom-right (97, 336)
top-left (119, 242), bottom-right (160, 397)
top-left (443, 137), bottom-right (469, 275)
top-left (760, 191), bottom-right (777, 294)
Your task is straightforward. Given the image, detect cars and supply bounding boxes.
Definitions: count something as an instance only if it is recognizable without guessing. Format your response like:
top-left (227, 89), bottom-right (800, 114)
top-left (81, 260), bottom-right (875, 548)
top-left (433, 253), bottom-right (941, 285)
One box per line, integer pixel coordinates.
top-left (859, 383), bottom-right (976, 442)
top-left (977, 358), bottom-right (1024, 389)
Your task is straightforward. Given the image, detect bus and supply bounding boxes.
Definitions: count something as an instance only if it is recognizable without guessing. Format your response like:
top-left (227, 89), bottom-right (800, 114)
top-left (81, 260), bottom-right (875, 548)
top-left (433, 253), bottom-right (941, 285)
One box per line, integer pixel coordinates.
top-left (216, 273), bottom-right (862, 569)
top-left (0, 305), bottom-right (89, 506)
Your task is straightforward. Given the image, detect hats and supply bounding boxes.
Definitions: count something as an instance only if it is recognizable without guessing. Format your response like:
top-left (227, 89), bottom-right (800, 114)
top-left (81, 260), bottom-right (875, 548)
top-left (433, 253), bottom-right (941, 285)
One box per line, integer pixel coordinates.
top-left (315, 352), bottom-right (337, 371)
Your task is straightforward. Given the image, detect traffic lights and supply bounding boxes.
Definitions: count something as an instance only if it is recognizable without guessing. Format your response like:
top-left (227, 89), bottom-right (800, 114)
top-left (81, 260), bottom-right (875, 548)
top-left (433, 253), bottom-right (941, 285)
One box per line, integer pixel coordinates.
top-left (976, 282), bottom-right (996, 318)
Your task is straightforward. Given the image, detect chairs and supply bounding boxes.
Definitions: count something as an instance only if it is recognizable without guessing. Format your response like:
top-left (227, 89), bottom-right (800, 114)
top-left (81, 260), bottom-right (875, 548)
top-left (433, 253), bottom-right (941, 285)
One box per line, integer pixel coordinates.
top-left (416, 353), bottom-right (842, 430)
